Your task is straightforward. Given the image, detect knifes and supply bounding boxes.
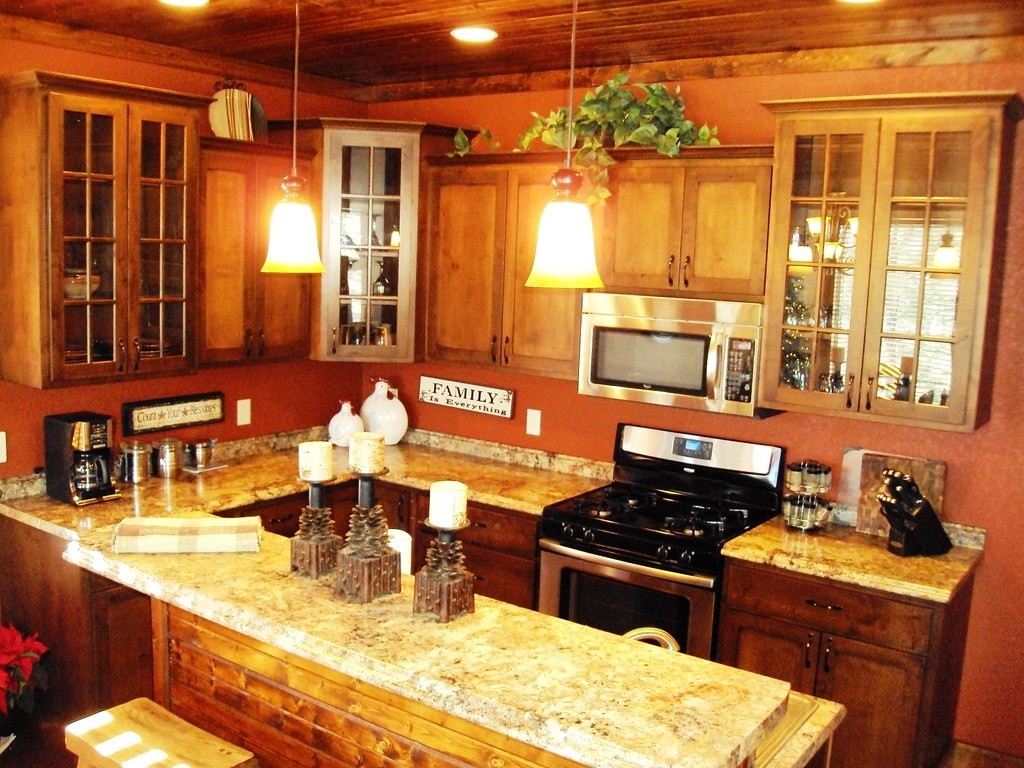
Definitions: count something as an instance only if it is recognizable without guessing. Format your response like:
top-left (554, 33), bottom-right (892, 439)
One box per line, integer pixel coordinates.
top-left (876, 468), bottom-right (923, 532)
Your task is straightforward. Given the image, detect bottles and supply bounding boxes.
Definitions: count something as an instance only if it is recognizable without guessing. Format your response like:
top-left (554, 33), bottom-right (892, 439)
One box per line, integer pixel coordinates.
top-left (328, 381), bottom-right (409, 446)
top-left (782, 459), bottom-right (832, 528)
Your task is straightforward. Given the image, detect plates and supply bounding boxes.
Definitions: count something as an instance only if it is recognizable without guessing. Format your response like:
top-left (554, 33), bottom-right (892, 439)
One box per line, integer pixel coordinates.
top-left (139, 337), bottom-right (171, 358)
top-left (64, 350), bottom-right (87, 360)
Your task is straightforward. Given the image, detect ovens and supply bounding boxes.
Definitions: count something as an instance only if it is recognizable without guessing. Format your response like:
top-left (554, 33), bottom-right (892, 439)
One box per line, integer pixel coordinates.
top-left (537, 538), bottom-right (716, 661)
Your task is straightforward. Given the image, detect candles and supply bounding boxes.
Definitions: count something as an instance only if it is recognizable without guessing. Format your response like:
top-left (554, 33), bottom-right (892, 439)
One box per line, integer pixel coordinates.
top-left (900, 355), bottom-right (913, 374)
top-left (299, 441), bottom-right (333, 481)
top-left (832, 348), bottom-right (845, 362)
top-left (430, 481), bottom-right (467, 528)
top-left (349, 432), bottom-right (385, 474)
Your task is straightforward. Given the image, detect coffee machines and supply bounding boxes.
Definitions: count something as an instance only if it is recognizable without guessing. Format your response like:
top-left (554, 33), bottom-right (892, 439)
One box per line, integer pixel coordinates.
top-left (43, 410), bottom-right (123, 506)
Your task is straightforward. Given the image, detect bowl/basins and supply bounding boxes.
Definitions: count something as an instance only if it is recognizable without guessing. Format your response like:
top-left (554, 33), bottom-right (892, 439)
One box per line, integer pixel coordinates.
top-left (64, 275), bottom-right (101, 300)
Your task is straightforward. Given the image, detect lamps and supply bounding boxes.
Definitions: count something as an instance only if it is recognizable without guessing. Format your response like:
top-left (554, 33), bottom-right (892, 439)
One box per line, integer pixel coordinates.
top-left (929, 179), bottom-right (963, 280)
top-left (260, 0), bottom-right (327, 275)
top-left (785, 133), bottom-right (856, 278)
top-left (524, 0), bottom-right (606, 290)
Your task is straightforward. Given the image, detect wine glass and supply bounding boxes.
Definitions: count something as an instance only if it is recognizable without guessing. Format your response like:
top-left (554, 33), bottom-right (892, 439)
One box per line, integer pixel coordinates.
top-left (364, 214), bottom-right (382, 246)
top-left (372, 261), bottom-right (394, 296)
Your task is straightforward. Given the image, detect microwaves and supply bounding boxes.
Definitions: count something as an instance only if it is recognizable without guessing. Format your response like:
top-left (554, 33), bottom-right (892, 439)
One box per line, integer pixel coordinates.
top-left (578, 291), bottom-right (763, 416)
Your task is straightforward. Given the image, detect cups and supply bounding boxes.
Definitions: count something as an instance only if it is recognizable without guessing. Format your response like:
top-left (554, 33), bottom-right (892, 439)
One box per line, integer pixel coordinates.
top-left (341, 320), bottom-right (391, 347)
top-left (120, 438), bottom-right (183, 482)
top-left (188, 437), bottom-right (212, 468)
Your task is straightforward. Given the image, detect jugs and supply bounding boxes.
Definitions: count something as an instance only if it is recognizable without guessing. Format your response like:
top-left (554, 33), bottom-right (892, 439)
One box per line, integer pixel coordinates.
top-left (74, 454), bottom-right (108, 489)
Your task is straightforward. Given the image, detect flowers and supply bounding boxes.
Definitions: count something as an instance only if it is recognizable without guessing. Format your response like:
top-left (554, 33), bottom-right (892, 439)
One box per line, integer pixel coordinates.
top-left (0, 621), bottom-right (50, 716)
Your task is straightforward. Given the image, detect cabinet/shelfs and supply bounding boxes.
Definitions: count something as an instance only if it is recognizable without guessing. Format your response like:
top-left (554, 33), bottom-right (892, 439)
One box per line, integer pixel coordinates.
top-left (754, 89), bottom-right (1024, 433)
top-left (211, 479), bottom-right (541, 612)
top-left (424, 146), bottom-right (775, 382)
top-left (714, 558), bottom-right (975, 768)
top-left (0, 70), bottom-right (480, 390)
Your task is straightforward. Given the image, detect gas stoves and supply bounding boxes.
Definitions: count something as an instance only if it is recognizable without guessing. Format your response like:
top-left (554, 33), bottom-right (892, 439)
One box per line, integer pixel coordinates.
top-left (540, 422), bottom-right (785, 576)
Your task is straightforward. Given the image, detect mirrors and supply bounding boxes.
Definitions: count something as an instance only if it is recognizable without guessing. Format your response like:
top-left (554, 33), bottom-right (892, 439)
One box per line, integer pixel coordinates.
top-left (209, 76), bottom-right (269, 146)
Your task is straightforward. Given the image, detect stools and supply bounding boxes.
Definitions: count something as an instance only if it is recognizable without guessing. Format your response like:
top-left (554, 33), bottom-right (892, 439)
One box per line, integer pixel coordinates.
top-left (64, 696), bottom-right (260, 768)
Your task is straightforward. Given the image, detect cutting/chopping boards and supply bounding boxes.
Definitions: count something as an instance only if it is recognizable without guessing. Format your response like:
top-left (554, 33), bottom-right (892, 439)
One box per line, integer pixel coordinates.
top-left (856, 453), bottom-right (947, 537)
